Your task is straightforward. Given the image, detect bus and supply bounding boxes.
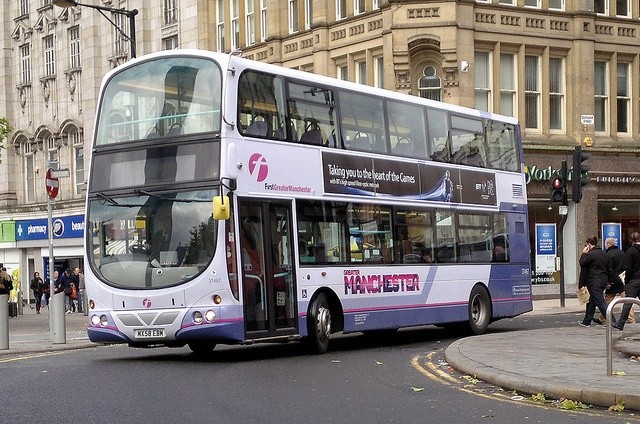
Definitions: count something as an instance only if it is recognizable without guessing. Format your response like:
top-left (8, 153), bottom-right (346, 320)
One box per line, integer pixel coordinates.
top-left (83, 49), bottom-right (532, 355)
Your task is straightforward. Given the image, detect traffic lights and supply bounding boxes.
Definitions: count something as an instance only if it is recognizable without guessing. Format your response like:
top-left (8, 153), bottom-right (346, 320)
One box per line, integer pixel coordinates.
top-left (549, 161), bottom-right (567, 206)
top-left (572, 145), bottom-right (591, 203)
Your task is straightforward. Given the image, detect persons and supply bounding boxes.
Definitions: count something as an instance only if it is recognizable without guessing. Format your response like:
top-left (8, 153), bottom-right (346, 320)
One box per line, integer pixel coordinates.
top-left (2, 259), bottom-right (84, 314)
top-left (151, 216), bottom-right (180, 255)
top-left (576, 232), bottom-right (639, 331)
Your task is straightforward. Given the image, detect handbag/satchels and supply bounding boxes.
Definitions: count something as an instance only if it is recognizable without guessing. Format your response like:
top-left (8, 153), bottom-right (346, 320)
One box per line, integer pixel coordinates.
top-left (65, 285), bottom-right (73, 295)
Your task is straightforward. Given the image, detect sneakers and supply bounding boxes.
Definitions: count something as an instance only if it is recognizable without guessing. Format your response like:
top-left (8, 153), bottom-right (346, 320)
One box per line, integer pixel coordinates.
top-left (577, 320), bottom-right (591, 328)
top-left (592, 317), bottom-right (602, 325)
top-left (611, 320), bottom-right (624, 330)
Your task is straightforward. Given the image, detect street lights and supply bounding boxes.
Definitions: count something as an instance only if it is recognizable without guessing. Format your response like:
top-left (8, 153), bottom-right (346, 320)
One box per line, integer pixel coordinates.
top-left (53, 1), bottom-right (138, 60)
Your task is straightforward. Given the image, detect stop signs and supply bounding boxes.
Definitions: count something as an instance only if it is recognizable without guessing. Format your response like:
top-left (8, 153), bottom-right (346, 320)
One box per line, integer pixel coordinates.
top-left (46, 169), bottom-right (59, 198)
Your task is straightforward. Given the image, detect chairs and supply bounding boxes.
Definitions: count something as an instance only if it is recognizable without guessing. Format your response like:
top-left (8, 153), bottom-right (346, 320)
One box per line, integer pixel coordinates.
top-left (274, 121), bottom-right (296, 141)
top-left (243, 115), bottom-right (269, 136)
top-left (325, 128), bottom-right (347, 147)
top-left (434, 144), bottom-right (448, 161)
top-left (391, 135), bottom-right (412, 155)
top-left (349, 129), bottom-right (371, 152)
top-left (299, 124), bottom-right (323, 144)
